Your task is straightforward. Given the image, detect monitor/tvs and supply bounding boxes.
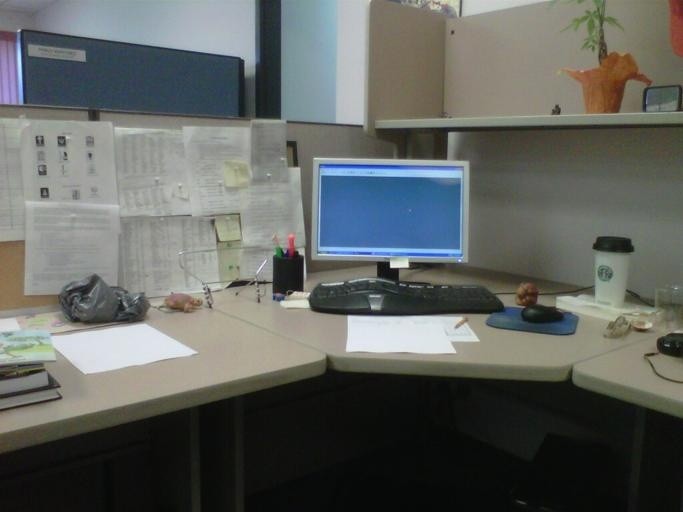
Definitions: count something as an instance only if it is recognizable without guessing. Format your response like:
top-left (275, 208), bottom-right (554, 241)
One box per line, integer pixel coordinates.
top-left (533, 269), bottom-right (664, 324)
top-left (0, 327), bottom-right (62, 411)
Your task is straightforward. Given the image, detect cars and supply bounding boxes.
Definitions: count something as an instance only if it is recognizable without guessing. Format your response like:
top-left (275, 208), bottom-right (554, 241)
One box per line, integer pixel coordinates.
top-left (653, 284), bottom-right (681, 321)
top-left (272, 253), bottom-right (305, 302)
top-left (590, 234), bottom-right (635, 307)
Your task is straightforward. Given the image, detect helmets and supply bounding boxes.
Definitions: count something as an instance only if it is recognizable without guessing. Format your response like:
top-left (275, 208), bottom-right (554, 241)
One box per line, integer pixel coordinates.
top-left (631, 319), bottom-right (653, 331)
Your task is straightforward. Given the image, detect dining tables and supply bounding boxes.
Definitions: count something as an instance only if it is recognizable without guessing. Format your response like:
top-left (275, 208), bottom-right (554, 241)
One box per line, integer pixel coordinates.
top-left (560, 0), bottom-right (652, 115)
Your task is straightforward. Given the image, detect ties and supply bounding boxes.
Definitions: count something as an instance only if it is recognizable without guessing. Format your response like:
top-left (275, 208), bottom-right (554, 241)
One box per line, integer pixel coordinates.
top-left (309, 157), bottom-right (470, 285)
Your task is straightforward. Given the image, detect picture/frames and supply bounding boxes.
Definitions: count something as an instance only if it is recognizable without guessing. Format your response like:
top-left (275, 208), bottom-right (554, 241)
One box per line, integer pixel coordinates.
top-left (0, 262), bottom-right (683, 454)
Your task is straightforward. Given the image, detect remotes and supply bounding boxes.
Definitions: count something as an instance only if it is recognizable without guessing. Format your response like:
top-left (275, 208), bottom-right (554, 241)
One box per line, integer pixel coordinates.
top-left (520, 304), bottom-right (563, 322)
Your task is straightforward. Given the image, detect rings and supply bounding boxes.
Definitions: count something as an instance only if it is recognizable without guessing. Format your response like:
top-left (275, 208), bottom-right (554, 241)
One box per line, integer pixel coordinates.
top-left (272, 234), bottom-right (299, 257)
top-left (454, 318), bottom-right (467, 329)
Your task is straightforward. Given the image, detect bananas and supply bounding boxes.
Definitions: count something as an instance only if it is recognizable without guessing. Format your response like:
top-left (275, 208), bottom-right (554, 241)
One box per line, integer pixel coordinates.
top-left (307, 283), bottom-right (506, 316)
